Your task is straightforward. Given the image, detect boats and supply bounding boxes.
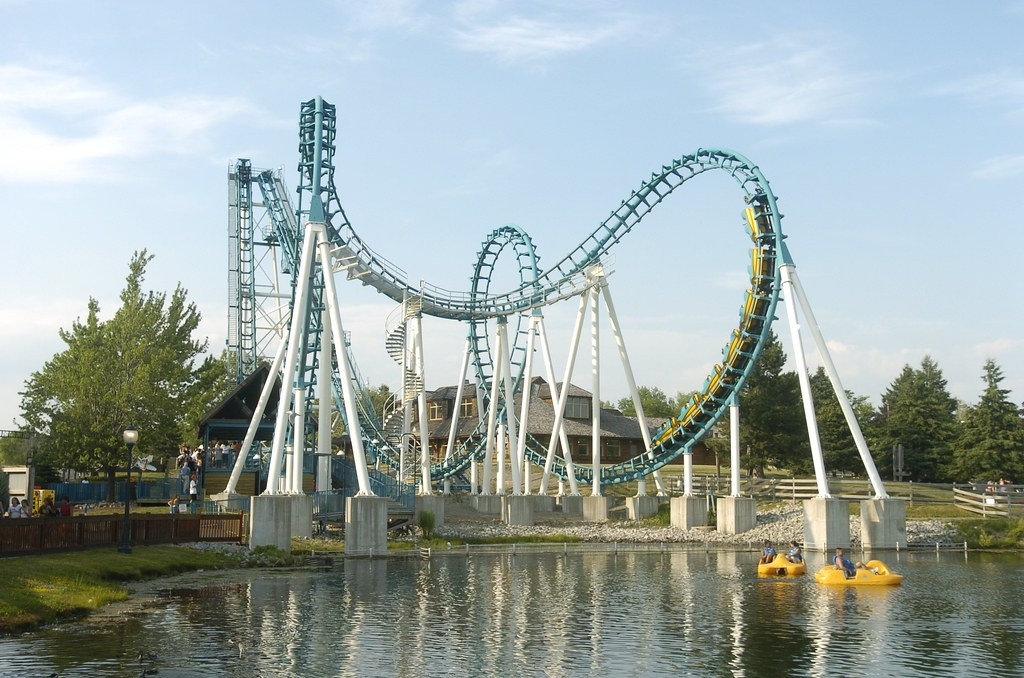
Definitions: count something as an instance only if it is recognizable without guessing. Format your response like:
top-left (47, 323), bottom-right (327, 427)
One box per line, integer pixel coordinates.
top-left (815, 560), bottom-right (903, 585)
top-left (758, 553), bottom-right (807, 575)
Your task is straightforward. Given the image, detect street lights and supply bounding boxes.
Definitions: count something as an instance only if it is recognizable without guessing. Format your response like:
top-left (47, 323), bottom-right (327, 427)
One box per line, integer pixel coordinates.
top-left (118, 422), bottom-right (139, 553)
top-left (398, 433), bottom-right (416, 495)
top-left (342, 424), bottom-right (349, 436)
top-left (362, 428), bottom-right (376, 467)
top-left (382, 436), bottom-right (400, 483)
top-left (371, 429), bottom-right (391, 477)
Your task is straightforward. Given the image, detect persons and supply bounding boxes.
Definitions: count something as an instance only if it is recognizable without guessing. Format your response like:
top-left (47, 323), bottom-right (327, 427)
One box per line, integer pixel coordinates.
top-left (168, 496), bottom-right (181, 513)
top-left (988, 478), bottom-right (994, 491)
top-left (999, 476), bottom-right (1006, 485)
top-left (0, 496), bottom-right (5, 520)
top-left (38, 498), bottom-right (56, 547)
top-left (176, 441), bottom-right (242, 471)
top-left (8, 497), bottom-right (28, 545)
top-left (761, 540), bottom-right (775, 563)
top-left (180, 462), bottom-right (191, 494)
top-left (833, 547), bottom-right (879, 574)
top-left (189, 475), bottom-right (197, 503)
top-left (786, 541), bottom-right (802, 563)
top-left (60, 497), bottom-right (73, 546)
top-left (21, 500), bottom-right (32, 546)
